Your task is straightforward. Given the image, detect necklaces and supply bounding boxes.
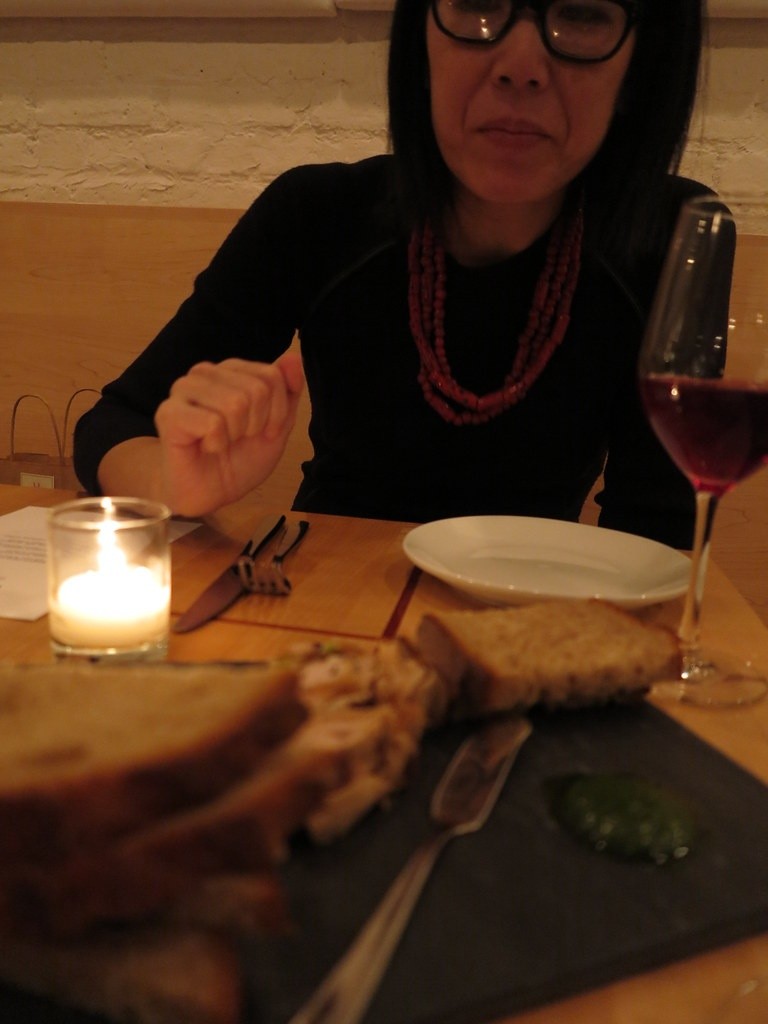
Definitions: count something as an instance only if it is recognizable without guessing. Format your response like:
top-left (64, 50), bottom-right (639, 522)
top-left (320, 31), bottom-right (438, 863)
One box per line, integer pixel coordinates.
top-left (406, 190), bottom-right (585, 426)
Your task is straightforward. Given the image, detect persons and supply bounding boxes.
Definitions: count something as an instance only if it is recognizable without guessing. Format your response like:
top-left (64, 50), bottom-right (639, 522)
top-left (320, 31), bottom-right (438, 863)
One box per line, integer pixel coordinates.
top-left (72, 0), bottom-right (736, 553)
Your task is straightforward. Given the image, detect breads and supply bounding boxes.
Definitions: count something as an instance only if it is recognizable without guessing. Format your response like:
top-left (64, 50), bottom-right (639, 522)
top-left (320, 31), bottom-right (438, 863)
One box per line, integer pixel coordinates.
top-left (1, 596), bottom-right (688, 1024)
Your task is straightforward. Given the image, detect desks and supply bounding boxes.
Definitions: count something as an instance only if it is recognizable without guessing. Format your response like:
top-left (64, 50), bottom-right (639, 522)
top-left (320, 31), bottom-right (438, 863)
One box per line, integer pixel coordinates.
top-left (1, 480), bottom-right (768, 1024)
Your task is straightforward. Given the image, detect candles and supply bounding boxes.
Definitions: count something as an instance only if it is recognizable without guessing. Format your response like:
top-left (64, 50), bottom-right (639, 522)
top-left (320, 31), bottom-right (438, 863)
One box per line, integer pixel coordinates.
top-left (46, 494), bottom-right (169, 668)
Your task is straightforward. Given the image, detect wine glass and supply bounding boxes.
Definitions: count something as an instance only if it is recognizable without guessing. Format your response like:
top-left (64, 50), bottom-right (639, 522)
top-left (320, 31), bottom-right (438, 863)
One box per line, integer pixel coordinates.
top-left (639, 197), bottom-right (768, 708)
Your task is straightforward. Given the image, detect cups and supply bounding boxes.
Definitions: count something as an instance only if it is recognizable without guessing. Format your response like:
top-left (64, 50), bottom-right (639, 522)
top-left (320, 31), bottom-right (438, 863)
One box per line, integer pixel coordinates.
top-left (47, 497), bottom-right (171, 667)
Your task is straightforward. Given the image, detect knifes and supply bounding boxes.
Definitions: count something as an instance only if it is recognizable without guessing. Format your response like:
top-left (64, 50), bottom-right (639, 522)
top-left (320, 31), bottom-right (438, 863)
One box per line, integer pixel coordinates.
top-left (286, 714), bottom-right (535, 1024)
top-left (170, 512), bottom-right (286, 651)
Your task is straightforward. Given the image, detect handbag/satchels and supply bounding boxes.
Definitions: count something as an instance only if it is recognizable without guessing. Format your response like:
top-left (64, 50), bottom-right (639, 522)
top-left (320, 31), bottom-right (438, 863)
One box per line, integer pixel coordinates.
top-left (0, 388), bottom-right (105, 494)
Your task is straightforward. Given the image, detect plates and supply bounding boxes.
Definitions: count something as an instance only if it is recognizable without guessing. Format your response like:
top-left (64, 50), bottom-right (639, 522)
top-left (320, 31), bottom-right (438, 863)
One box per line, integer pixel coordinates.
top-left (400, 516), bottom-right (691, 607)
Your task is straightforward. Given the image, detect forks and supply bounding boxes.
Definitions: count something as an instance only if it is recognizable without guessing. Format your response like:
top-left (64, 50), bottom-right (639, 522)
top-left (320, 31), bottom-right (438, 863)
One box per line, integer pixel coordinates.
top-left (239, 520), bottom-right (309, 595)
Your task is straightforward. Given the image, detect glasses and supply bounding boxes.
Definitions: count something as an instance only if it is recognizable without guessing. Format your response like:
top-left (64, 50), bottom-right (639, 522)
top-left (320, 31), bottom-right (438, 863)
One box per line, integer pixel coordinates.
top-left (430, 0), bottom-right (639, 64)
